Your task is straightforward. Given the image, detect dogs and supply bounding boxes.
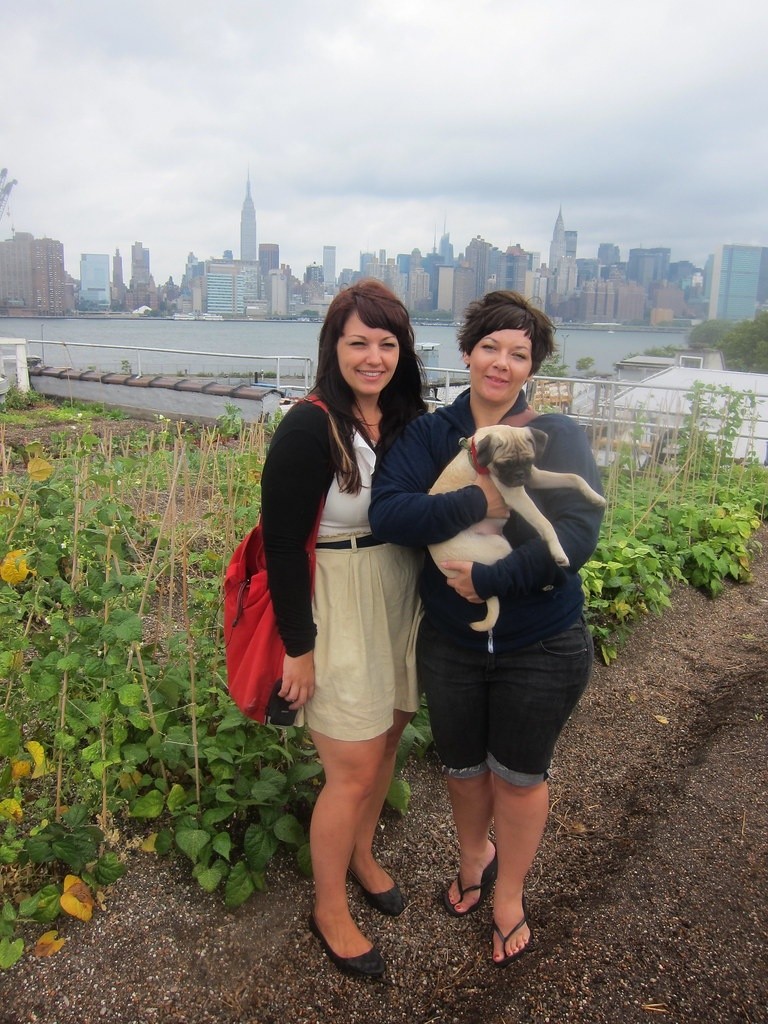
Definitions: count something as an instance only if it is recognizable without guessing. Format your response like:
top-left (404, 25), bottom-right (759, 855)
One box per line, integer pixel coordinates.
top-left (426, 419), bottom-right (606, 632)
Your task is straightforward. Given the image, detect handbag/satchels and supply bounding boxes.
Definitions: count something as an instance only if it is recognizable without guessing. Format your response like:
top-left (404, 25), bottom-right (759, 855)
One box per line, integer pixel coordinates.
top-left (224, 525), bottom-right (328, 726)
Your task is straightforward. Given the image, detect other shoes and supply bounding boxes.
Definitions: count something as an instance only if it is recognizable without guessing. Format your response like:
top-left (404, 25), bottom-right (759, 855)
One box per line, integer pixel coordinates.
top-left (347, 864), bottom-right (404, 916)
top-left (309, 907), bottom-right (385, 976)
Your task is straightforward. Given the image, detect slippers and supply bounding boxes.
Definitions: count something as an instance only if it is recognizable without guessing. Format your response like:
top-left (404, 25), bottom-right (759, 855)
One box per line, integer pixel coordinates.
top-left (491, 893), bottom-right (533, 968)
top-left (443, 839), bottom-right (498, 916)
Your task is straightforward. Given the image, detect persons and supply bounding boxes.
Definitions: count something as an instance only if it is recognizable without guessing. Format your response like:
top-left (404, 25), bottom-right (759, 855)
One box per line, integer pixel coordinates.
top-left (367, 291), bottom-right (605, 968)
top-left (265, 280), bottom-right (432, 980)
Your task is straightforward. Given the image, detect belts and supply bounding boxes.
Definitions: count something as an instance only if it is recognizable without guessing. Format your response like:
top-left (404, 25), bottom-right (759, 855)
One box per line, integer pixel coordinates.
top-left (315, 534), bottom-right (392, 549)
